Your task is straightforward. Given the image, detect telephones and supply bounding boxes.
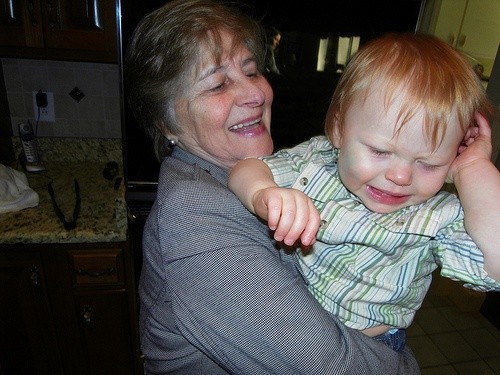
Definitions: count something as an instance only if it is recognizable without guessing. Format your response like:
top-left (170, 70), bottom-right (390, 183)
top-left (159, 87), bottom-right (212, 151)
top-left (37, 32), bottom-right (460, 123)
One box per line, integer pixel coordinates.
top-left (18, 121), bottom-right (47, 172)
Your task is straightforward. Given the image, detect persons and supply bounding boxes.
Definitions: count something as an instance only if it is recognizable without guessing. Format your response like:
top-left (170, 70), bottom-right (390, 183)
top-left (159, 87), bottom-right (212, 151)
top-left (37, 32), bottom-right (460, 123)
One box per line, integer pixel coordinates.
top-left (228, 33), bottom-right (500, 356)
top-left (133, 0), bottom-right (419, 375)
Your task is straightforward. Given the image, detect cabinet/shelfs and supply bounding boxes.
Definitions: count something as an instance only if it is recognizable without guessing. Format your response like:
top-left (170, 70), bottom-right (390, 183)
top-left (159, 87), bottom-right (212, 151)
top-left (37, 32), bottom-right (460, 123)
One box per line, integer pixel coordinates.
top-left (1, 136), bottom-right (141, 375)
top-left (0, 0), bottom-right (119, 63)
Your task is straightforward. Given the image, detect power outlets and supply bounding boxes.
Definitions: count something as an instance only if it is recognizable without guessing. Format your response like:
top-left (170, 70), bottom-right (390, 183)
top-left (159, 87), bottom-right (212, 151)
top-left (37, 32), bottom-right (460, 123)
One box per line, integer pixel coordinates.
top-left (33, 92), bottom-right (55, 122)
